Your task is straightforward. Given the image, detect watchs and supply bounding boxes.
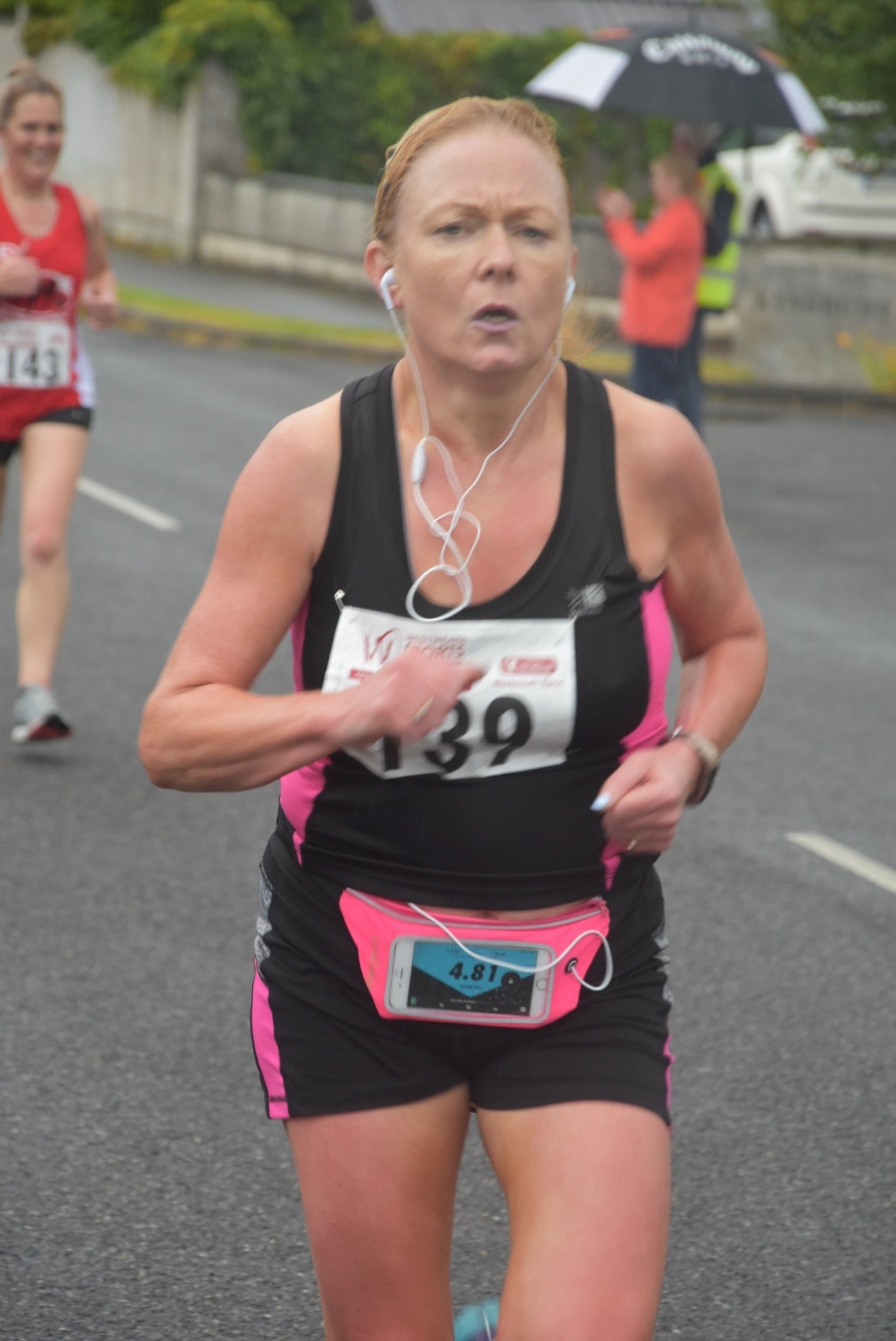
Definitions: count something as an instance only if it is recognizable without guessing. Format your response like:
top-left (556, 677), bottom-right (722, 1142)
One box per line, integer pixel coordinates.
top-left (661, 726), bottom-right (720, 807)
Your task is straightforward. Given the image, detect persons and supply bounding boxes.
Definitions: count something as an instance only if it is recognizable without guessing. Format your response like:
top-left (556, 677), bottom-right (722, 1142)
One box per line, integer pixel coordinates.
top-left (137, 92), bottom-right (768, 1340)
top-left (0, 59), bottom-right (117, 741)
top-left (671, 125), bottom-right (754, 430)
top-left (598, 151), bottom-right (705, 428)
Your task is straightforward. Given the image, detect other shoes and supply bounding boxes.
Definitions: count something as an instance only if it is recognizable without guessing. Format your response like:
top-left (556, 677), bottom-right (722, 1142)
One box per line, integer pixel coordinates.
top-left (8, 687), bottom-right (72, 747)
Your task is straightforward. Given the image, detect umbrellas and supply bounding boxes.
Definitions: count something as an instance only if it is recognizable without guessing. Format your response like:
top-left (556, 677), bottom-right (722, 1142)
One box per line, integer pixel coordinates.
top-left (520, 23), bottom-right (829, 138)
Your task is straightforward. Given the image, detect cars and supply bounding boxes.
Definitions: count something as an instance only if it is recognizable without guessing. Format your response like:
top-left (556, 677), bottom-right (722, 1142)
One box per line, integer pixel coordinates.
top-left (713, 122), bottom-right (895, 249)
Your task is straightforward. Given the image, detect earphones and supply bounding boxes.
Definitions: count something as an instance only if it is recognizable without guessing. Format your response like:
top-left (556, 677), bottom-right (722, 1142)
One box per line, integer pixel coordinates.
top-left (561, 276), bottom-right (578, 308)
top-left (381, 268), bottom-right (399, 309)
